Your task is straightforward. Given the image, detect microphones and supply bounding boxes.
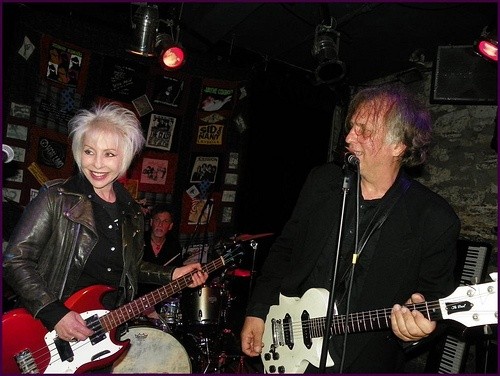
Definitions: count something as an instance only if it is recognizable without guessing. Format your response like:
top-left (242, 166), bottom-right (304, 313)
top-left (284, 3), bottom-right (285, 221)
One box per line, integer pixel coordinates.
top-left (344, 153), bottom-right (360, 166)
top-left (209, 191), bottom-right (222, 204)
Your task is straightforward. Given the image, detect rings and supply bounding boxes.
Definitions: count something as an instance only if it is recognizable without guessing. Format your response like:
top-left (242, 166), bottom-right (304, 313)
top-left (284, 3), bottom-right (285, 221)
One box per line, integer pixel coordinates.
top-left (71, 337), bottom-right (78, 342)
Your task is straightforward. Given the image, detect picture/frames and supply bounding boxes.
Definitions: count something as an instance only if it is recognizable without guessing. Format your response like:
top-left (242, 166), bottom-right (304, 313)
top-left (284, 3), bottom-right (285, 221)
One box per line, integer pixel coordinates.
top-left (429, 42), bottom-right (498, 105)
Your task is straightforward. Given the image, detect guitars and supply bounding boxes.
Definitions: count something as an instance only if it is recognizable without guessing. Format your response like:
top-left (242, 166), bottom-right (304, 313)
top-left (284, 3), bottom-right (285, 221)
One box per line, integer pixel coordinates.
top-left (1, 243), bottom-right (246, 374)
top-left (261, 272), bottom-right (499, 373)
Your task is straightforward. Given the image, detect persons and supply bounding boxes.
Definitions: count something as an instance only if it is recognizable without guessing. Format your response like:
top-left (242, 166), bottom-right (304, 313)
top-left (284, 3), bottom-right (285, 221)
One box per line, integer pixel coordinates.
top-left (144, 206), bottom-right (183, 268)
top-left (241, 88), bottom-right (460, 374)
top-left (2, 100), bottom-right (208, 373)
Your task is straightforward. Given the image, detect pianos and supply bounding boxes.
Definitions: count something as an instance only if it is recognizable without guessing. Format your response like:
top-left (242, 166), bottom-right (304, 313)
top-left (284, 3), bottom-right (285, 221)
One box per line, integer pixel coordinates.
top-left (425, 239), bottom-right (492, 374)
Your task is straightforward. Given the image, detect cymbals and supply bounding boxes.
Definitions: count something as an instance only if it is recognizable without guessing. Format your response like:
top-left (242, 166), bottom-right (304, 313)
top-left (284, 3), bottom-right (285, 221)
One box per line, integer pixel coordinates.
top-left (236, 233), bottom-right (271, 240)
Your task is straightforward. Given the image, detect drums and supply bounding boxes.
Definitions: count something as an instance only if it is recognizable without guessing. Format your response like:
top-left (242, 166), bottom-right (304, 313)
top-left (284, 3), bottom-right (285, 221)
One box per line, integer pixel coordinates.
top-left (182, 282), bottom-right (222, 326)
top-left (155, 298), bottom-right (184, 325)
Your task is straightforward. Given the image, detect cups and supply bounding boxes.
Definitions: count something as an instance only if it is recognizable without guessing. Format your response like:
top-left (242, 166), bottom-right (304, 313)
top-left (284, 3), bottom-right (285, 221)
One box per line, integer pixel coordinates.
top-left (162, 299), bottom-right (178, 323)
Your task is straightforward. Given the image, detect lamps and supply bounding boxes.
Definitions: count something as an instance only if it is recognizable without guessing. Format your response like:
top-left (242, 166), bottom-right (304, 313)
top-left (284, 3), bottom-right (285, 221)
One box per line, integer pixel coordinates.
top-left (156, 25), bottom-right (185, 69)
top-left (313, 17), bottom-right (347, 82)
top-left (125, 3), bottom-right (161, 56)
top-left (473, 25), bottom-right (500, 62)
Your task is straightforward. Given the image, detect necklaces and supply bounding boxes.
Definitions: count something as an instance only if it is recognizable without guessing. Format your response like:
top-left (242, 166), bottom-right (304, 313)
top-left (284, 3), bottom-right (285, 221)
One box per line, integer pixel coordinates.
top-left (151, 238), bottom-right (165, 250)
